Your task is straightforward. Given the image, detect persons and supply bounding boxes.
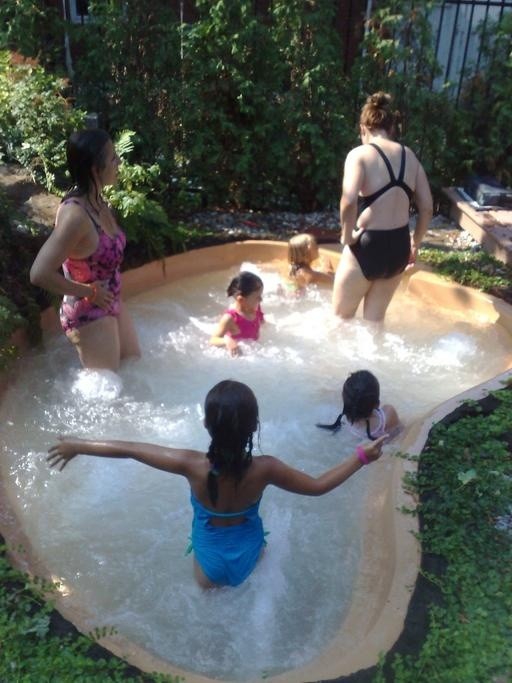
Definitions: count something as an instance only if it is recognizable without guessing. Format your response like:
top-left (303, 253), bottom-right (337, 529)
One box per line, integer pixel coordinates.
top-left (210, 271), bottom-right (267, 357)
top-left (46, 380), bottom-right (389, 589)
top-left (317, 369), bottom-right (402, 442)
top-left (334, 91), bottom-right (433, 322)
top-left (30, 128), bottom-right (141, 373)
top-left (274, 234), bottom-right (335, 297)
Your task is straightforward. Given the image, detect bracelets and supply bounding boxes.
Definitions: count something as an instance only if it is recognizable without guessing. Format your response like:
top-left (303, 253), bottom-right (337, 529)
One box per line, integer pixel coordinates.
top-left (355, 446), bottom-right (369, 465)
top-left (85, 282), bottom-right (98, 304)
